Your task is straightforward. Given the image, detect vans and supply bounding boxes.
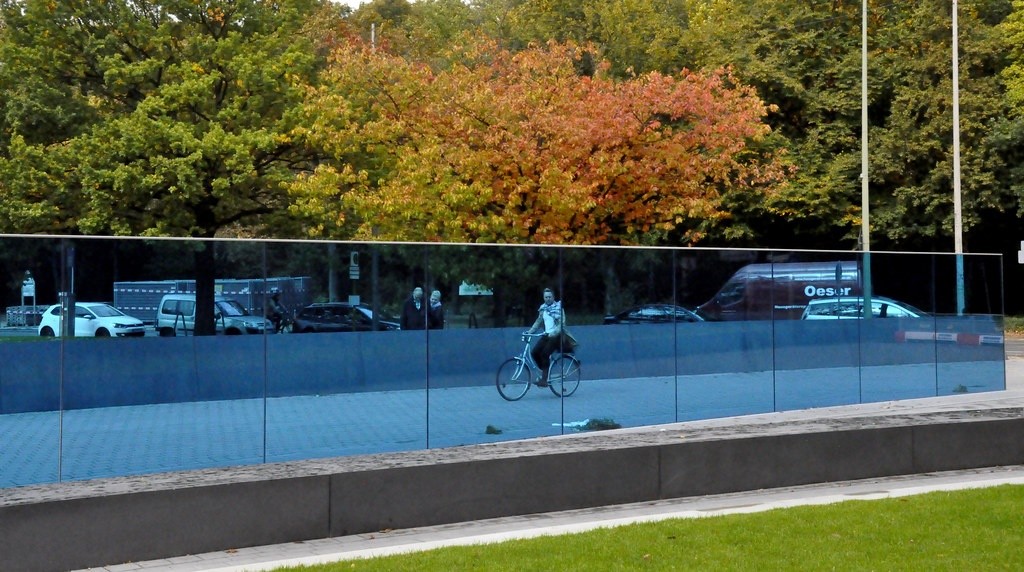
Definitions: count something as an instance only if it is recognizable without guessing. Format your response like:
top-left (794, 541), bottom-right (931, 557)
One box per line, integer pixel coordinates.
top-left (690, 261), bottom-right (861, 319)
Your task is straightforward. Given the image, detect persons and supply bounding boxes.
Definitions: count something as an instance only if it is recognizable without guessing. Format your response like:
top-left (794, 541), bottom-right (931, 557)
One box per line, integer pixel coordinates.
top-left (398, 286), bottom-right (444, 330)
top-left (265, 286), bottom-right (289, 331)
top-left (525, 287), bottom-right (565, 387)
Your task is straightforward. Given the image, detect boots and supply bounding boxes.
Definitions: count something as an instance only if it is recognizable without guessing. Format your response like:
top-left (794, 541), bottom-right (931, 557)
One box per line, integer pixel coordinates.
top-left (538, 367), bottom-right (548, 387)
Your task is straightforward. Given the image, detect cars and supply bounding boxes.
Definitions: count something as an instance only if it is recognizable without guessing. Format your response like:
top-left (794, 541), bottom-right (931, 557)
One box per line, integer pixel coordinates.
top-left (802, 295), bottom-right (931, 320)
top-left (602, 304), bottom-right (706, 325)
top-left (38, 302), bottom-right (146, 339)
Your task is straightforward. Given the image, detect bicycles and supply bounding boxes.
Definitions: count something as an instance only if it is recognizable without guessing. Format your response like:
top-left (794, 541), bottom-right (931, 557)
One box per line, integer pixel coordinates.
top-left (496, 331), bottom-right (582, 401)
top-left (264, 310), bottom-right (294, 333)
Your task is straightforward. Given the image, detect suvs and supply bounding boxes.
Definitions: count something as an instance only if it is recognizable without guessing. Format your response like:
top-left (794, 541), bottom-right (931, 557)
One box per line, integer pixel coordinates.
top-left (292, 301), bottom-right (400, 334)
top-left (155, 292), bottom-right (276, 336)
